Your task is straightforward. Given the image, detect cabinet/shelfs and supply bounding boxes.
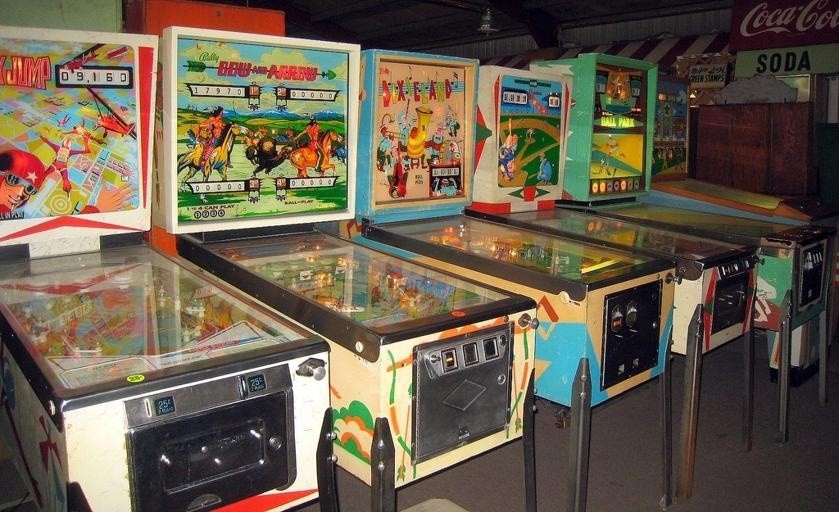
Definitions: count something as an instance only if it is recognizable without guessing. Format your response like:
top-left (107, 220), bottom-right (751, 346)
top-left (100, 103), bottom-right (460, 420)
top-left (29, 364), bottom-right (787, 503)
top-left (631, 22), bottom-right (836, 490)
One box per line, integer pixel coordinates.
top-left (340, 47), bottom-right (683, 512)
top-left (528, 53), bottom-right (837, 444)
top-left (651, 72), bottom-right (839, 388)
top-left (142, 26), bottom-right (540, 511)
top-left (696, 101), bottom-right (817, 196)
top-left (0, 26), bottom-right (340, 512)
top-left (465, 64), bottom-right (765, 501)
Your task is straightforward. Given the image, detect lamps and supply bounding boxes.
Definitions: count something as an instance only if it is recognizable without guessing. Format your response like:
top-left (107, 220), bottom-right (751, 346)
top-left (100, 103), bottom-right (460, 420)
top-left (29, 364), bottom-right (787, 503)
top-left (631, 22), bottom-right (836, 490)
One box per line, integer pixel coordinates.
top-left (473, 6), bottom-right (502, 40)
